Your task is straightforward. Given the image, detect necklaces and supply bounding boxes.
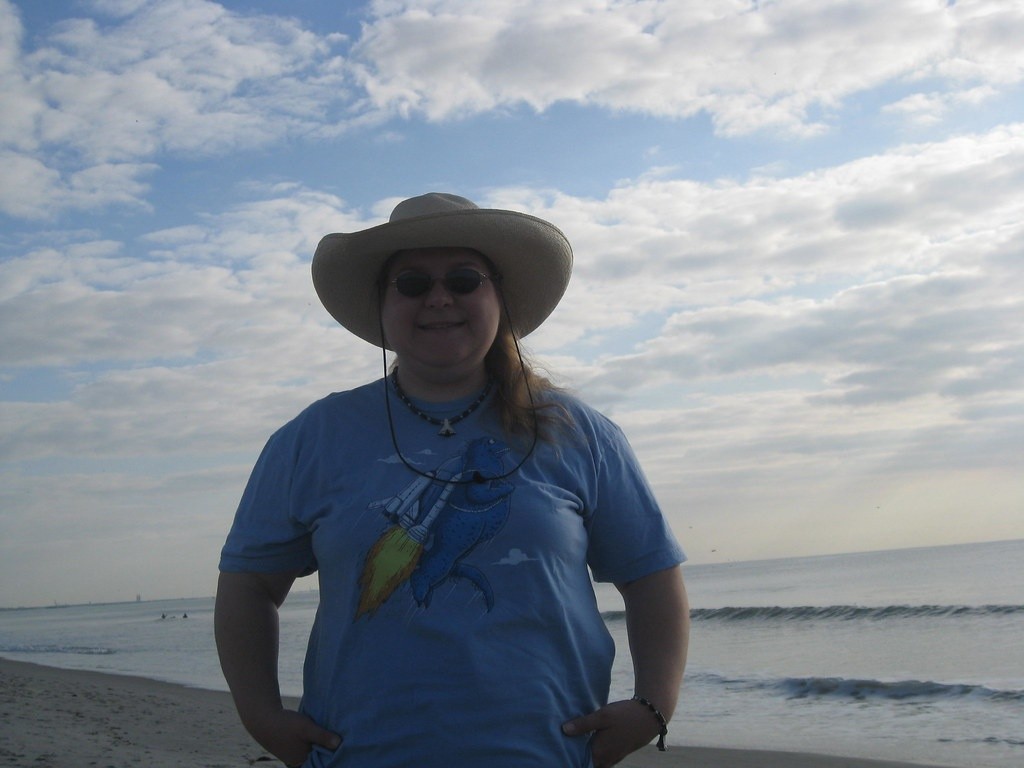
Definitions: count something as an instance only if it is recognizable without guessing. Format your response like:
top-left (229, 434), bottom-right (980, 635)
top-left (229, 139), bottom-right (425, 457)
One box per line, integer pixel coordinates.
top-left (389, 363), bottom-right (499, 438)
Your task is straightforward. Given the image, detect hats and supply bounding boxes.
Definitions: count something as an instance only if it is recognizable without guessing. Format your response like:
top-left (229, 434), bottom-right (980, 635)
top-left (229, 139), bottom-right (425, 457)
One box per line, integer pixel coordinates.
top-left (311, 192), bottom-right (574, 351)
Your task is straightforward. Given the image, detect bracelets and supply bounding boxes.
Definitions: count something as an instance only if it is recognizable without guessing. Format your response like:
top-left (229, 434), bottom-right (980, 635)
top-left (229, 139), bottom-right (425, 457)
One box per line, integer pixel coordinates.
top-left (632, 695), bottom-right (671, 752)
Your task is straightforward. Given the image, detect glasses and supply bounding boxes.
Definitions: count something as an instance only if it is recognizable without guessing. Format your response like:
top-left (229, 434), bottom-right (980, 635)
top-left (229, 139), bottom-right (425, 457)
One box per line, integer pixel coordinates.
top-left (385, 271), bottom-right (493, 296)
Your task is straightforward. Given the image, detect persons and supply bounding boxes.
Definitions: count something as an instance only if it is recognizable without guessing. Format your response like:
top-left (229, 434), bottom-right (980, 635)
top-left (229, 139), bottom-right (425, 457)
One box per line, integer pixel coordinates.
top-left (213, 188), bottom-right (691, 768)
